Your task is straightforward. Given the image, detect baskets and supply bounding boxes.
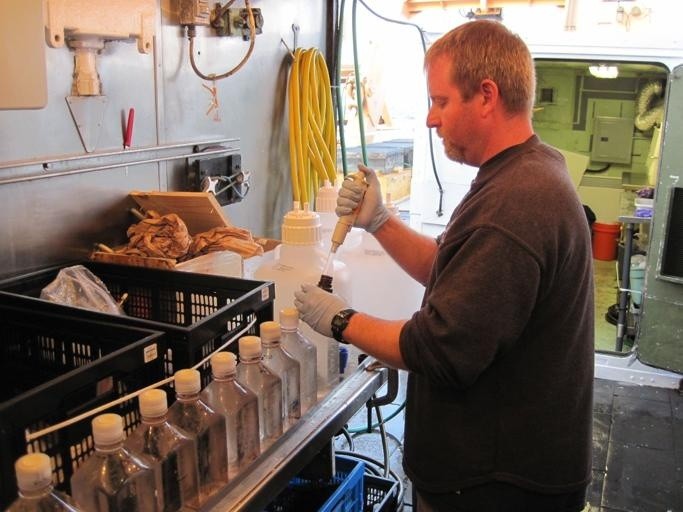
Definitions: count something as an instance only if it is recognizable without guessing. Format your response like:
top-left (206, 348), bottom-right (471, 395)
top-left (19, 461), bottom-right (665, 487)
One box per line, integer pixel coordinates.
top-left (0, 256), bottom-right (276, 510)
top-left (265, 454), bottom-right (398, 512)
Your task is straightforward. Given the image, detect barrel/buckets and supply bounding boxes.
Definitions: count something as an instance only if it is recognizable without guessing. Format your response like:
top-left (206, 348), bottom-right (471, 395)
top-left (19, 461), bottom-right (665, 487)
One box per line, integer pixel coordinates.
top-left (591, 222), bottom-right (620, 262)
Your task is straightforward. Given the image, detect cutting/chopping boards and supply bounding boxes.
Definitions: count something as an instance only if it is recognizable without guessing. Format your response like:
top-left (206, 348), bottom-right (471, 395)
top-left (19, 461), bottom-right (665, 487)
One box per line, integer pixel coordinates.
top-left (129, 191), bottom-right (232, 236)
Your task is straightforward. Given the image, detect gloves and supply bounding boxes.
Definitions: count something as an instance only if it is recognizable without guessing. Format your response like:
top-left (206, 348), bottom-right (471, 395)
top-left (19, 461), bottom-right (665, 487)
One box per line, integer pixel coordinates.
top-left (294, 282), bottom-right (351, 339)
top-left (335, 163), bottom-right (390, 234)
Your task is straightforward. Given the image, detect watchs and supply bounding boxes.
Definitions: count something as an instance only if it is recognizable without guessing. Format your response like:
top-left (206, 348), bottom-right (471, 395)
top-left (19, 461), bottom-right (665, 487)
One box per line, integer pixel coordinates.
top-left (332, 310), bottom-right (355, 344)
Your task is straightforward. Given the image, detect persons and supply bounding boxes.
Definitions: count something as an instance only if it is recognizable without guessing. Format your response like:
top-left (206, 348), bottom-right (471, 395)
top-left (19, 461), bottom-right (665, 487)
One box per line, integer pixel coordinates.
top-left (295, 20), bottom-right (593, 510)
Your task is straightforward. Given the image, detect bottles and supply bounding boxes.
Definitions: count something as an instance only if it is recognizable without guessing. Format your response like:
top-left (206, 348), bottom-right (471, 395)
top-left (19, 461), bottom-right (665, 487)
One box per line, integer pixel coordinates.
top-left (260, 321), bottom-right (301, 435)
top-left (69, 413), bottom-right (165, 512)
top-left (126, 388), bottom-right (200, 512)
top-left (5, 453), bottom-right (81, 512)
top-left (279, 309), bottom-right (318, 417)
top-left (235, 336), bottom-right (284, 454)
top-left (166, 369), bottom-right (229, 509)
top-left (200, 351), bottom-right (261, 479)
top-left (299, 319), bottom-right (340, 404)
